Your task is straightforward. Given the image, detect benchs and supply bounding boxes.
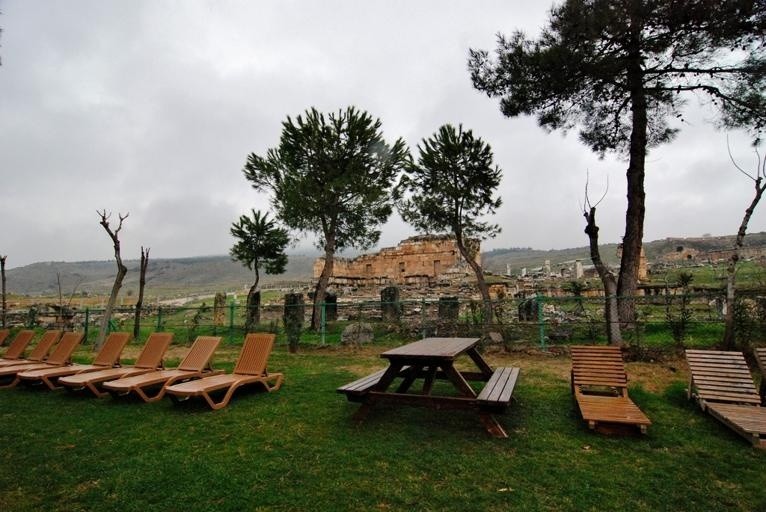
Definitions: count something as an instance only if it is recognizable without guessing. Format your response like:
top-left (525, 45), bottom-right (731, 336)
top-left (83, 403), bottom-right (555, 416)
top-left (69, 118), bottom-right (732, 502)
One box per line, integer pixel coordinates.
top-left (335, 337), bottom-right (519, 438)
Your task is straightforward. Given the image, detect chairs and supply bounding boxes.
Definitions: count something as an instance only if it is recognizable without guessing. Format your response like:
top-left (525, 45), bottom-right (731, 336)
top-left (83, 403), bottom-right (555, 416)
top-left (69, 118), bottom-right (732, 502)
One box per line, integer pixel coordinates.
top-left (0, 328), bottom-right (285, 410)
top-left (570, 344), bottom-right (766, 450)
